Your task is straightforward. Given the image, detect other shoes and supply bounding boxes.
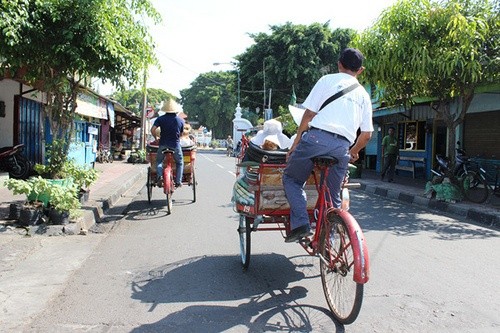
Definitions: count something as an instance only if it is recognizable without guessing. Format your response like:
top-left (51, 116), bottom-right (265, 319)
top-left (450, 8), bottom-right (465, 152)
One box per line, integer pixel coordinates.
top-left (389, 179), bottom-right (395, 183)
top-left (157, 177), bottom-right (163, 186)
top-left (285, 225), bottom-right (310, 243)
top-left (175, 181), bottom-right (182, 186)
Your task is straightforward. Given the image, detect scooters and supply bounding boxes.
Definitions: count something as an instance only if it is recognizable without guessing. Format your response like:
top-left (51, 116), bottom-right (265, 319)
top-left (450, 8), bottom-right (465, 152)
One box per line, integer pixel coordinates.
top-left (0, 143), bottom-right (32, 181)
top-left (429, 142), bottom-right (481, 190)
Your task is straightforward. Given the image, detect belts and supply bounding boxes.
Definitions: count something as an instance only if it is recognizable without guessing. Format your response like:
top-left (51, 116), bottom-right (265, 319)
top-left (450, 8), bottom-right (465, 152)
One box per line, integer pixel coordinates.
top-left (313, 127), bottom-right (348, 141)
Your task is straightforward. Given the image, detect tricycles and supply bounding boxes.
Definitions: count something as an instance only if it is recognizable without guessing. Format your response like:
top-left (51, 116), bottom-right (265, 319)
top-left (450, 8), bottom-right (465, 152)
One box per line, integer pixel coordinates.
top-left (145, 140), bottom-right (198, 215)
top-left (232, 134), bottom-right (370, 325)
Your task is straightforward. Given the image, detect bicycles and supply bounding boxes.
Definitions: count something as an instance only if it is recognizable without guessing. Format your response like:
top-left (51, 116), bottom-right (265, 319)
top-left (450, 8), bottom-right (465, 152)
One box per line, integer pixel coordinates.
top-left (459, 151), bottom-right (500, 204)
top-left (226, 143), bottom-right (234, 158)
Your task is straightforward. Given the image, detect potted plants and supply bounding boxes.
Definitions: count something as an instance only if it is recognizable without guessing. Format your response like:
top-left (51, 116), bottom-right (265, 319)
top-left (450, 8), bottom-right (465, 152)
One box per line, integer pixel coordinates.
top-left (2, 163), bottom-right (105, 226)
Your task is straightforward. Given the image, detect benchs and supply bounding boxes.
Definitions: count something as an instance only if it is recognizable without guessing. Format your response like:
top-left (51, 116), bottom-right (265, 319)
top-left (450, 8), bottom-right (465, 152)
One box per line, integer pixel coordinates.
top-left (395, 157), bottom-right (426, 180)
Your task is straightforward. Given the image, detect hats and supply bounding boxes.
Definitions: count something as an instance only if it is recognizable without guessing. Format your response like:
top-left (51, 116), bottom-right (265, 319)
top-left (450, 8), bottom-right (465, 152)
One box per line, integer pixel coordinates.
top-left (340, 48), bottom-right (362, 72)
top-left (250, 119), bottom-right (291, 150)
top-left (161, 98), bottom-right (182, 114)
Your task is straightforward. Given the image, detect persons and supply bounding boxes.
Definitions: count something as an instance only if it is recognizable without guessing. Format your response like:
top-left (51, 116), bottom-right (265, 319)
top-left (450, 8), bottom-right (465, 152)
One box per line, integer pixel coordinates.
top-left (380, 128), bottom-right (400, 182)
top-left (226, 135), bottom-right (233, 153)
top-left (283, 48), bottom-right (375, 261)
top-left (151, 99), bottom-right (184, 187)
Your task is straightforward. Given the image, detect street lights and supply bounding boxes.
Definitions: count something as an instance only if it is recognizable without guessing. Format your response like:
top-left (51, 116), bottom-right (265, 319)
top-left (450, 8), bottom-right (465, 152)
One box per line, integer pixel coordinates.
top-left (213, 62), bottom-right (240, 106)
